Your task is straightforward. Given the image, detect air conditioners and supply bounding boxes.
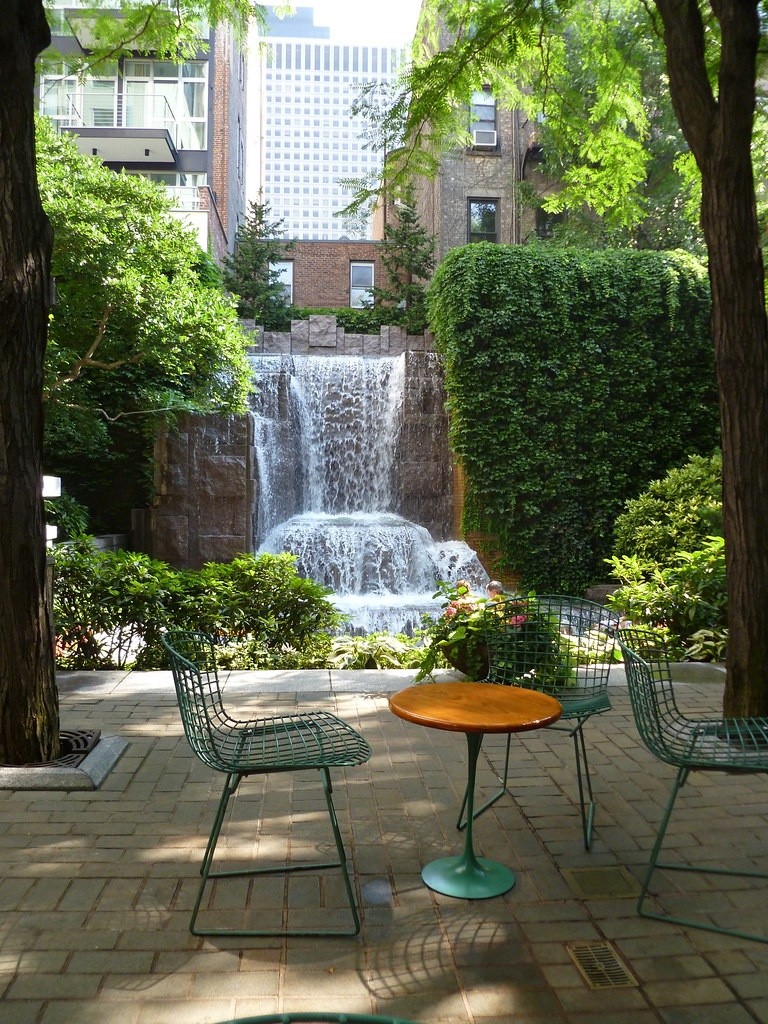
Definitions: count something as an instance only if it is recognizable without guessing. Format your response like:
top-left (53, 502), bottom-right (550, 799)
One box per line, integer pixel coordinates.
top-left (470, 129), bottom-right (497, 152)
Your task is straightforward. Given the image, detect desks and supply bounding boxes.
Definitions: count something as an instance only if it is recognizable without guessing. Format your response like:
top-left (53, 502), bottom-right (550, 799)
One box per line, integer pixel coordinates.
top-left (388, 679), bottom-right (563, 900)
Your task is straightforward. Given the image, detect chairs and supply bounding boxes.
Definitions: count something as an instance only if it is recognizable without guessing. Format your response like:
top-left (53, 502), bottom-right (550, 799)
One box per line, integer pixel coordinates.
top-left (613, 628), bottom-right (768, 944)
top-left (456, 592), bottom-right (621, 860)
top-left (159, 628), bottom-right (371, 949)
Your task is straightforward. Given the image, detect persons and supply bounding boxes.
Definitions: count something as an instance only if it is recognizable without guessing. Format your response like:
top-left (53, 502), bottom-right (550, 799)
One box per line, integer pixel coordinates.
top-left (486, 581), bottom-right (514, 599)
top-left (454, 581), bottom-right (477, 604)
